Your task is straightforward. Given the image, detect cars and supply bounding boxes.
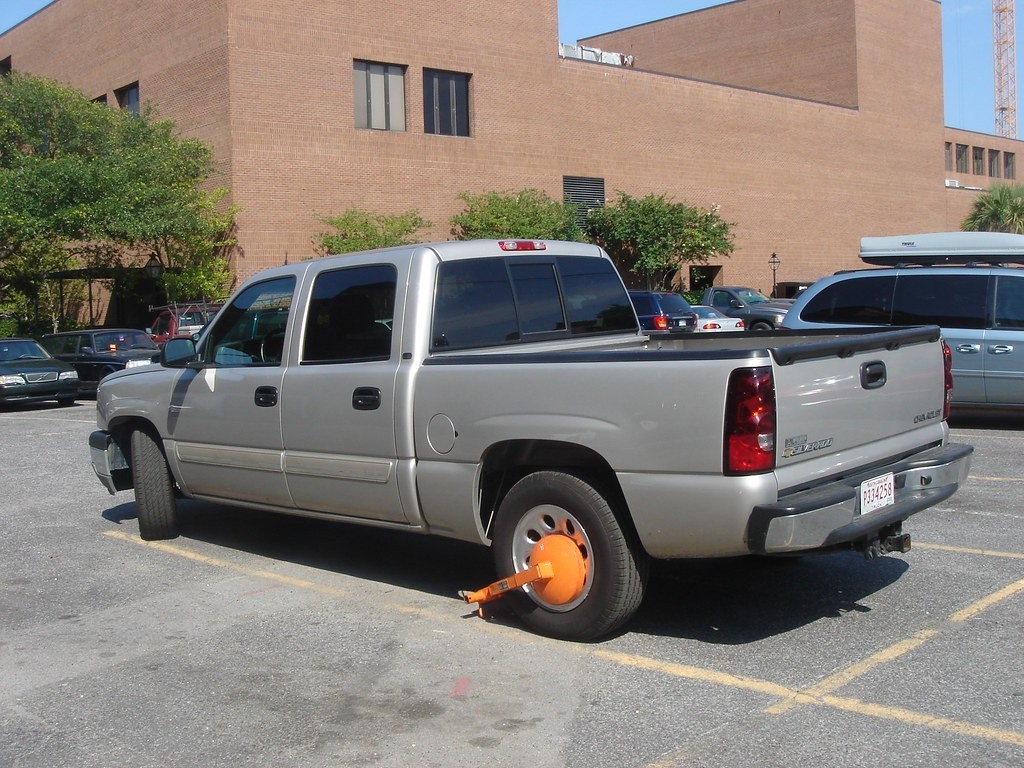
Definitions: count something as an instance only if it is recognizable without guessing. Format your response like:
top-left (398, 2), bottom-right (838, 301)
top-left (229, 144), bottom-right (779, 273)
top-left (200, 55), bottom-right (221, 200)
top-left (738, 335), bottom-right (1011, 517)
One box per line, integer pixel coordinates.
top-left (0, 337), bottom-right (81, 409)
top-left (41, 328), bottom-right (164, 394)
top-left (691, 306), bottom-right (744, 333)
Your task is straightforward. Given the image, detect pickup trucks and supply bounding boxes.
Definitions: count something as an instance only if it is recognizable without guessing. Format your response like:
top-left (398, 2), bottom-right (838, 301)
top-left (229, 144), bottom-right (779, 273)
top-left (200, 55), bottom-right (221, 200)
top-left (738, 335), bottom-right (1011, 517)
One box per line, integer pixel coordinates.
top-left (87, 238), bottom-right (975, 642)
top-left (692, 286), bottom-right (793, 331)
top-left (121, 304), bottom-right (228, 346)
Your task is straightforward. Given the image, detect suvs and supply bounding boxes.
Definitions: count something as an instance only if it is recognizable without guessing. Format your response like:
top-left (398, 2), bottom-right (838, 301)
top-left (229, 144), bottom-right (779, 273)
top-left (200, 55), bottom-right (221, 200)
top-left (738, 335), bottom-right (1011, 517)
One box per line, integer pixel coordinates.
top-left (770, 281), bottom-right (816, 299)
top-left (626, 290), bottom-right (698, 336)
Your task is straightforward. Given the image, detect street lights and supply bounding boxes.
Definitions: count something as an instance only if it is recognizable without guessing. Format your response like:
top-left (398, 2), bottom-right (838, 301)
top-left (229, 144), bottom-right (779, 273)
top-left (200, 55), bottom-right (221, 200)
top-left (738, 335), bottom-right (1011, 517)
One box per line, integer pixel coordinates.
top-left (768, 252), bottom-right (780, 299)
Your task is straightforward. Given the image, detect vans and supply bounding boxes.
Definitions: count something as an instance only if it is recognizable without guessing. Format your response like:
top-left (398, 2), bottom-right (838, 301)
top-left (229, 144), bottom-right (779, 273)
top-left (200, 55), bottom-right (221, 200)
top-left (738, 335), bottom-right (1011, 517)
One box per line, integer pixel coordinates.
top-left (779, 230), bottom-right (1023, 418)
